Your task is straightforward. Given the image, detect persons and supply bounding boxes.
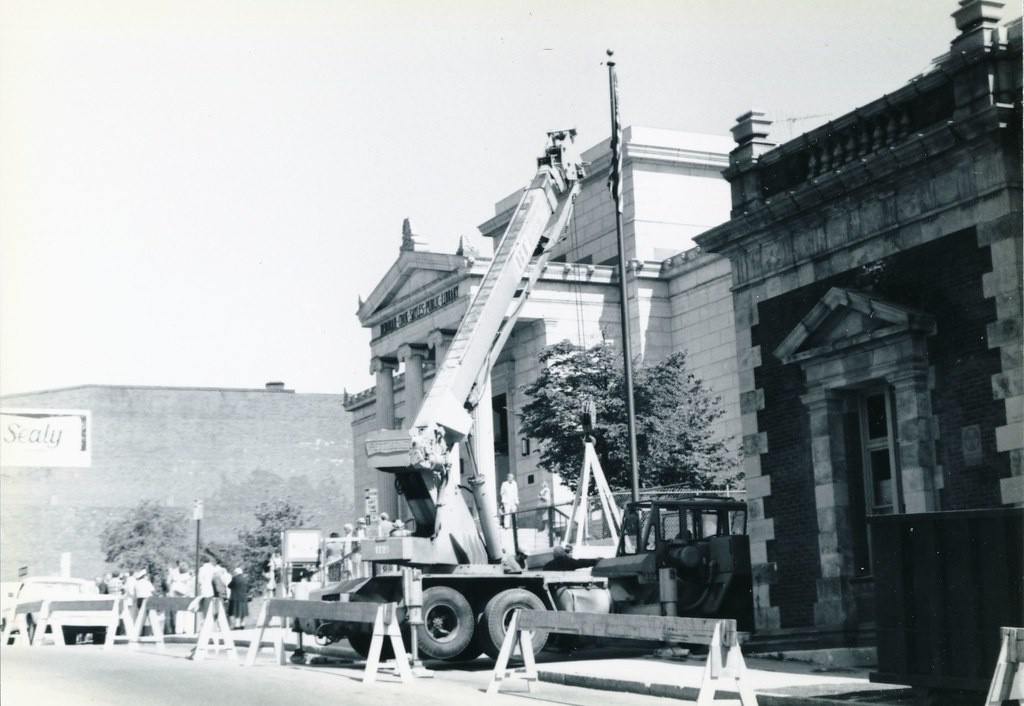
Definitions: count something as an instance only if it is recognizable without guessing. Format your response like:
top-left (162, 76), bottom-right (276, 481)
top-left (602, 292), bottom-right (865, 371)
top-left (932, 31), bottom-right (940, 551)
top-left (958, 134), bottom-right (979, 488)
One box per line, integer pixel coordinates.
top-left (326, 531), bottom-right (343, 582)
top-left (540, 544), bottom-right (604, 571)
top-left (500, 472), bottom-right (518, 528)
top-left (378, 511), bottom-right (411, 571)
top-left (228, 567), bottom-right (249, 630)
top-left (261, 548), bottom-right (281, 601)
top-left (212, 559), bottom-right (231, 600)
top-left (95, 569), bottom-right (163, 637)
top-left (197, 556), bottom-right (216, 598)
top-left (353, 517), bottom-right (369, 577)
top-left (536, 480), bottom-right (551, 530)
top-left (340, 522), bottom-right (357, 579)
top-left (165, 557), bottom-right (195, 634)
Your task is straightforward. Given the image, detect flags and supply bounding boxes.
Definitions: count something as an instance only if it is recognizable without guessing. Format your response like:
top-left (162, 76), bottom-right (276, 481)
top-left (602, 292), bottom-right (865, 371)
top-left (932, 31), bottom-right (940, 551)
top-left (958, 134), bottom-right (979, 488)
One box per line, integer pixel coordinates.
top-left (607, 75), bottom-right (624, 214)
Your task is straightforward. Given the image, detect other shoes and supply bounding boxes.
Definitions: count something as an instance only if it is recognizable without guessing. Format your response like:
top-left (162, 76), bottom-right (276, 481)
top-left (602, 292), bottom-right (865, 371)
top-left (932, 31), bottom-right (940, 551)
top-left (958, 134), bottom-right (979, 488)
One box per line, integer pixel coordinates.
top-left (232, 626), bottom-right (244, 630)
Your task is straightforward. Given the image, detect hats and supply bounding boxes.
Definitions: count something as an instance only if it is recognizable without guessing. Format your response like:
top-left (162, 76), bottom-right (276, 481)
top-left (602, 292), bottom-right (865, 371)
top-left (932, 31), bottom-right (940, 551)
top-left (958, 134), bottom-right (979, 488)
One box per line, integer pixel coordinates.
top-left (357, 518), bottom-right (366, 524)
top-left (138, 568), bottom-right (147, 579)
top-left (393, 519), bottom-right (404, 528)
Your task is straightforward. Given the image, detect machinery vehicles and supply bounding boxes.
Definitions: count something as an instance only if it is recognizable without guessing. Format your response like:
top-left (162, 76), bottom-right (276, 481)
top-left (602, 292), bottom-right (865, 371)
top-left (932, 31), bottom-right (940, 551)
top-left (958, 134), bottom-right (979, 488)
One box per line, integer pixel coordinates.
top-left (296, 127), bottom-right (756, 664)
